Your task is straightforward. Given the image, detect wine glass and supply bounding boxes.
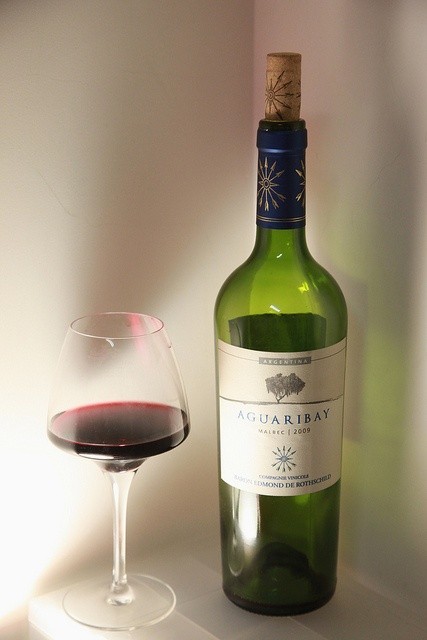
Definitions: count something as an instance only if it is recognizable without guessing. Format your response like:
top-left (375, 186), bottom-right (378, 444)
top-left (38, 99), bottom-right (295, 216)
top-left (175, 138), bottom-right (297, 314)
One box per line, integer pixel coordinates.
top-left (50, 311), bottom-right (191, 629)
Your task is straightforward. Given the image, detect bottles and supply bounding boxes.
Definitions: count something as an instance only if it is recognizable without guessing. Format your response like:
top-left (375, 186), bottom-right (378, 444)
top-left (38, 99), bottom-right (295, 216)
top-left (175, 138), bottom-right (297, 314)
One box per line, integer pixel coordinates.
top-left (215, 52), bottom-right (349, 617)
